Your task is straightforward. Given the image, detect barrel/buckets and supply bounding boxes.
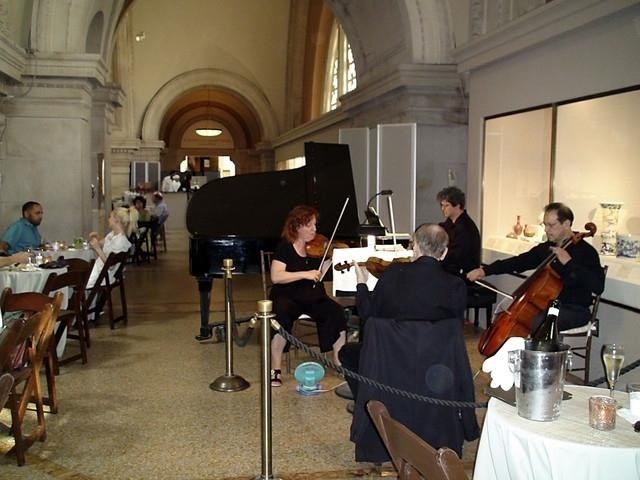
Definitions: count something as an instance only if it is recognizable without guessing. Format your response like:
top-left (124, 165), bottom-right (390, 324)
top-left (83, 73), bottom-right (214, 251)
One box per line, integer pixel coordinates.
top-left (505, 337), bottom-right (572, 422)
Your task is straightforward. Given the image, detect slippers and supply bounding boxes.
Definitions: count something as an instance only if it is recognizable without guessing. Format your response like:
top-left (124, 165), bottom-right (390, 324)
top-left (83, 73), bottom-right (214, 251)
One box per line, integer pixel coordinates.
top-left (270, 368), bottom-right (284, 388)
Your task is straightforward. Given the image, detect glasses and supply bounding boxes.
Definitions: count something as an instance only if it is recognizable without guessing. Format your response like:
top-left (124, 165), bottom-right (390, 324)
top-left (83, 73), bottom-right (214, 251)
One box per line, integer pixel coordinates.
top-left (543, 221), bottom-right (560, 227)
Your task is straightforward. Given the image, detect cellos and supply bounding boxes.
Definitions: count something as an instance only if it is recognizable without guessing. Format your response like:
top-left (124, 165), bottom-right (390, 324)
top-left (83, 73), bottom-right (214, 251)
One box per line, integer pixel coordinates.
top-left (479, 222), bottom-right (596, 357)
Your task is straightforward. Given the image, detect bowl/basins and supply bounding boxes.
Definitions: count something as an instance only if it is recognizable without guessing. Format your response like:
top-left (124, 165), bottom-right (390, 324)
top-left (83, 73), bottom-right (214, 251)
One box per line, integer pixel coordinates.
top-left (614, 232), bottom-right (640, 261)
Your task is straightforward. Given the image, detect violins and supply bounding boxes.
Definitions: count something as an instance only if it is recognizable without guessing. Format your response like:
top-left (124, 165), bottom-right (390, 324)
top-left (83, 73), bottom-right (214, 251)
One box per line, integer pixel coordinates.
top-left (305, 235), bottom-right (349, 258)
top-left (334, 257), bottom-right (415, 278)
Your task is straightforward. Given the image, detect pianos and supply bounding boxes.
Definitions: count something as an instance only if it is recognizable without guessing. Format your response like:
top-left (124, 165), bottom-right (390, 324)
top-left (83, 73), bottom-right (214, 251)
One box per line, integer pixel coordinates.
top-left (187, 142), bottom-right (411, 340)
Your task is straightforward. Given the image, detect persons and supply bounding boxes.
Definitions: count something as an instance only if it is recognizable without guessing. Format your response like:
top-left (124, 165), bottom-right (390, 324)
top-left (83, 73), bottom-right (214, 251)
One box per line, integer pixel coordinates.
top-left (79, 206), bottom-right (139, 291)
top-left (134, 197), bottom-right (152, 262)
top-left (149, 191), bottom-right (168, 230)
top-left (466, 201), bottom-right (605, 344)
top-left (0, 251), bottom-right (36, 269)
top-left (267, 205), bottom-right (350, 388)
top-left (0, 202), bottom-right (53, 256)
top-left (432, 186), bottom-right (480, 282)
top-left (161, 167), bottom-right (193, 193)
top-left (333, 225), bottom-right (465, 397)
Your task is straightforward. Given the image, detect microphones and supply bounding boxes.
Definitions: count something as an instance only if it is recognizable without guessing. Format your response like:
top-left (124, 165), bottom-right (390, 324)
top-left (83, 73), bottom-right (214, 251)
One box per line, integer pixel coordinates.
top-left (368, 190), bottom-right (392, 224)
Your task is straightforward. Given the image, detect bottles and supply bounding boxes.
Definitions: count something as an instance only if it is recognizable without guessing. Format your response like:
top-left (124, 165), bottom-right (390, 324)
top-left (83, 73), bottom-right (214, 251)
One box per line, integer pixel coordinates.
top-left (514, 215), bottom-right (523, 235)
top-left (600, 201), bottom-right (622, 257)
top-left (530, 299), bottom-right (562, 351)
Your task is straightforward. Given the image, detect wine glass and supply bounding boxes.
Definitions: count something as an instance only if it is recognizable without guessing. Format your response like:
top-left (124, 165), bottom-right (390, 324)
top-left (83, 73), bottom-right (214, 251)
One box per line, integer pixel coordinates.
top-left (603, 344), bottom-right (625, 411)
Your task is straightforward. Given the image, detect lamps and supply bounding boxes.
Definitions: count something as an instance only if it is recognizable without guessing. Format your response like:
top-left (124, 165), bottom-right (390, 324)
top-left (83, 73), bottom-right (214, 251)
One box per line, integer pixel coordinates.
top-left (194, 87), bottom-right (223, 136)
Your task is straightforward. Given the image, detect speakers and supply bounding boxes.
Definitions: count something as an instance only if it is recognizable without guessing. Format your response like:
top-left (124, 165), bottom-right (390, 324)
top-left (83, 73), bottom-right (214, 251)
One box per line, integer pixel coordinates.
top-left (147, 161), bottom-right (160, 191)
top-left (131, 161), bottom-right (147, 191)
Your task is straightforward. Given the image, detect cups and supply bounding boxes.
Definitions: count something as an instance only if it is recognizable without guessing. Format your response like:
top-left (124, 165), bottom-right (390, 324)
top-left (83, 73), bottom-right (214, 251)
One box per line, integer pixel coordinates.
top-left (367, 235), bottom-right (376, 257)
top-left (628, 382), bottom-right (640, 418)
top-left (588, 395), bottom-right (619, 431)
top-left (25, 237), bottom-right (89, 271)
